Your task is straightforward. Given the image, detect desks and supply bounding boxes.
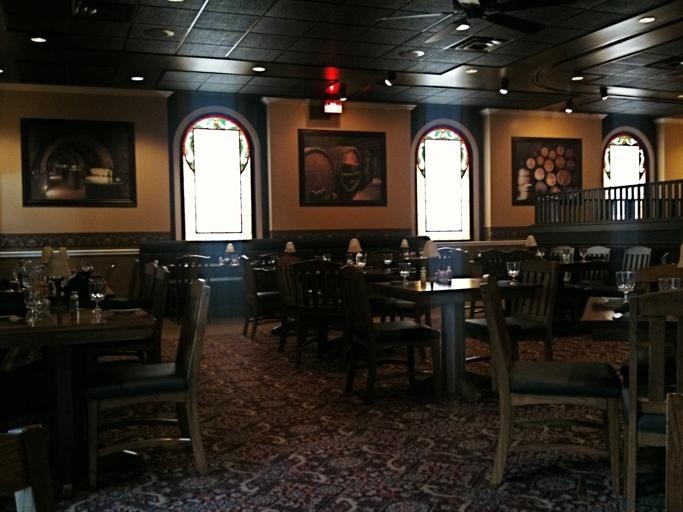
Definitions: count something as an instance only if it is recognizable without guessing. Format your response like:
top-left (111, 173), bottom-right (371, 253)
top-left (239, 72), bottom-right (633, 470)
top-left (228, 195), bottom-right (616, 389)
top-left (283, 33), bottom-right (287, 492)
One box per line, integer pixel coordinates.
top-left (167, 257), bottom-right (266, 314)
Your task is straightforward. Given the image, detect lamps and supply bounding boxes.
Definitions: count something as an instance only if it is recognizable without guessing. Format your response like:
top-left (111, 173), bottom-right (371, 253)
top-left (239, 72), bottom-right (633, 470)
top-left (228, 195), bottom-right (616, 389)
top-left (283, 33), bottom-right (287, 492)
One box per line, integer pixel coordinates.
top-left (499, 76), bottom-right (509, 95)
top-left (563, 98), bottom-right (575, 114)
top-left (600, 87), bottom-right (608, 101)
top-left (384, 70), bottom-right (400, 87)
top-left (339, 84), bottom-right (347, 102)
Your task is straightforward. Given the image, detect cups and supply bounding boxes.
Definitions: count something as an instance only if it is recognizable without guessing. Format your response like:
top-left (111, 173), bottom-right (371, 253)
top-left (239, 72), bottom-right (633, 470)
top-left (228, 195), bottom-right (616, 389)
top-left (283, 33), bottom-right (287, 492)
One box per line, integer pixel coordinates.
top-left (21, 286), bottom-right (45, 312)
top-left (356, 250), bottom-right (393, 268)
top-left (658, 277), bottom-right (680, 291)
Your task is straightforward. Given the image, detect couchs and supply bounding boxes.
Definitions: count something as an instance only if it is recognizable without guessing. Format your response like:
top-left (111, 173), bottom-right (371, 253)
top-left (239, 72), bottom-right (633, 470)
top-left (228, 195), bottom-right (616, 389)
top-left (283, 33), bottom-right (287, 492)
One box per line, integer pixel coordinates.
top-left (138, 234), bottom-right (437, 296)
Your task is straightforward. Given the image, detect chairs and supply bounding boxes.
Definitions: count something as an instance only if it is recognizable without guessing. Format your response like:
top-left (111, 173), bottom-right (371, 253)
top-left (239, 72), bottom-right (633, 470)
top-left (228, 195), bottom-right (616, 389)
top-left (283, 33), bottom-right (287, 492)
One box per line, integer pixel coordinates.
top-left (470, 259), bottom-right (682, 512)
top-left (1, 251), bottom-right (219, 494)
top-left (238, 247), bottom-right (653, 400)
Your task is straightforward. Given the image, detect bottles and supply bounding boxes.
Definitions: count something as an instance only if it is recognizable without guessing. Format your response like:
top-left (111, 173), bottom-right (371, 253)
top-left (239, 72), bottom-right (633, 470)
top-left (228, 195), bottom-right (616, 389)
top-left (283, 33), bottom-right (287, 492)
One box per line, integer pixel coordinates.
top-left (218, 256), bottom-right (222, 265)
top-left (419, 267), bottom-right (428, 286)
top-left (445, 265), bottom-right (452, 285)
top-left (69, 290), bottom-right (79, 311)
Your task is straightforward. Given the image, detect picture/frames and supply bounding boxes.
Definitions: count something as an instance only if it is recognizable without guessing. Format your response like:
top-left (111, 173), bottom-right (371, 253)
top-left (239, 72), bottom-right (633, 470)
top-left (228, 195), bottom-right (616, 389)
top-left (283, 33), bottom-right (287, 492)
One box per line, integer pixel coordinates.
top-left (21, 118), bottom-right (136, 207)
top-left (512, 136), bottom-right (582, 205)
top-left (298, 129), bottom-right (387, 207)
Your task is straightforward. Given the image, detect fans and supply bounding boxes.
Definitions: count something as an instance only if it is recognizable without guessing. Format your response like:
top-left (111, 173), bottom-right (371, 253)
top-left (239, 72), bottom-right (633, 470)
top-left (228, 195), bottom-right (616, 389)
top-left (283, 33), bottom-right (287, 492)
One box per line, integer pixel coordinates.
top-left (425, 0), bottom-right (575, 44)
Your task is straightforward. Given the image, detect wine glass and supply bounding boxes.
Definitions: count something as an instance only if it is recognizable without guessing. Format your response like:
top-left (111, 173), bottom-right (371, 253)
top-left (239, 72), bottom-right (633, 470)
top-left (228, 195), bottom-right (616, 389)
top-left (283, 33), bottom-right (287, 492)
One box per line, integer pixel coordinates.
top-left (535, 246), bottom-right (588, 263)
top-left (88, 275), bottom-right (106, 315)
top-left (223, 252), bottom-right (238, 266)
top-left (504, 261), bottom-right (520, 286)
top-left (396, 261), bottom-right (412, 285)
top-left (615, 271), bottom-right (635, 302)
